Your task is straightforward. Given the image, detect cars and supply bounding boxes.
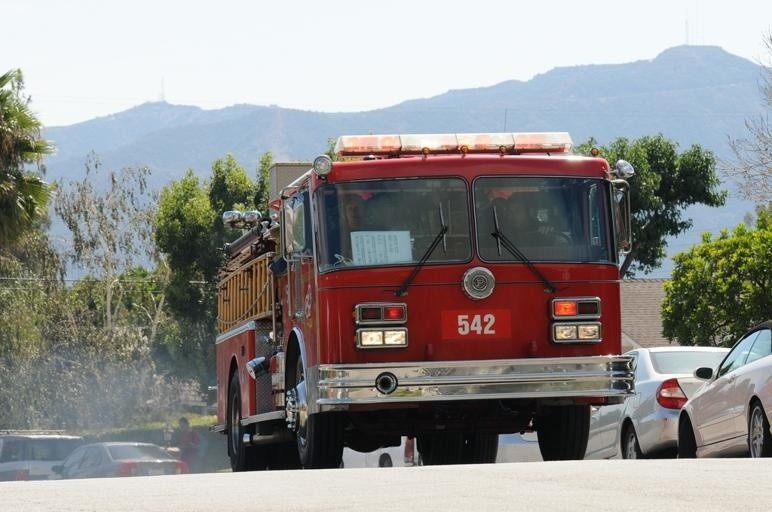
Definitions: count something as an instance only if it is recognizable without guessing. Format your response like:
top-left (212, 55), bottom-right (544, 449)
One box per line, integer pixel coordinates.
top-left (677, 319), bottom-right (772, 458)
top-left (48, 443), bottom-right (192, 479)
top-left (583, 346), bottom-right (760, 460)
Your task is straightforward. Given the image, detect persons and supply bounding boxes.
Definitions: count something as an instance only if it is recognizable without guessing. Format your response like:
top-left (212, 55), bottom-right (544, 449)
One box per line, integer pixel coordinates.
top-left (503, 201), bottom-right (560, 248)
top-left (340, 194), bottom-right (370, 259)
top-left (171, 418), bottom-right (202, 473)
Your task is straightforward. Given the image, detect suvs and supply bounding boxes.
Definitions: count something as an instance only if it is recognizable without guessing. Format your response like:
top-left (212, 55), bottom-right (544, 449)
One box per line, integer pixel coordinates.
top-left (0, 435), bottom-right (84, 479)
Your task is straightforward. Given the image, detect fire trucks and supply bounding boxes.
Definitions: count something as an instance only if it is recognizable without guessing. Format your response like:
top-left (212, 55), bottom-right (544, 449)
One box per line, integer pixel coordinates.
top-left (210, 131), bottom-right (636, 471)
